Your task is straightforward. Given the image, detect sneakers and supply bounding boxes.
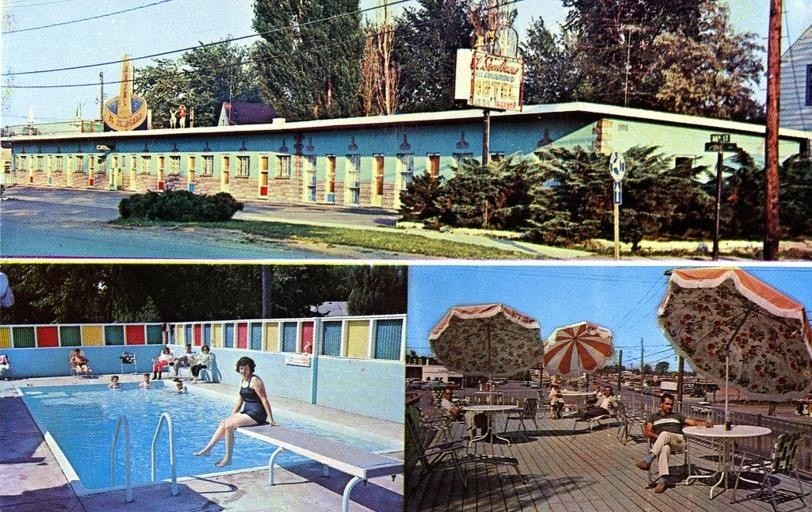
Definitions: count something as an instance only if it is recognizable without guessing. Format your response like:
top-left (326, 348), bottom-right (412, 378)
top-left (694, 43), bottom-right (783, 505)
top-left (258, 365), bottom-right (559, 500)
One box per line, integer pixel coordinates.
top-left (635, 460), bottom-right (650, 470)
top-left (655, 482), bottom-right (667, 493)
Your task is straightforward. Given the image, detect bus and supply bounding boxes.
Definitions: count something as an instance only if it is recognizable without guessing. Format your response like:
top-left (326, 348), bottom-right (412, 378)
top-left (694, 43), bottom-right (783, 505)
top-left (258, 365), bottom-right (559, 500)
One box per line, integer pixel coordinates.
top-left (490, 378), bottom-right (508, 385)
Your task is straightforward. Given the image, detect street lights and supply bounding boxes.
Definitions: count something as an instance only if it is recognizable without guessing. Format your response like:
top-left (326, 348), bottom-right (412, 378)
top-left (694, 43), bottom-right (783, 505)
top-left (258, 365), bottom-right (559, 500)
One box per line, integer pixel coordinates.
top-left (98, 71), bottom-right (107, 122)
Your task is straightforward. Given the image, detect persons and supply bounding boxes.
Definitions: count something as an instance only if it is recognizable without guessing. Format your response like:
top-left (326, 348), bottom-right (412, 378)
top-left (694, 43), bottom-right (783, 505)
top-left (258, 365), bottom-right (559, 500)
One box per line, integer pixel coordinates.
top-left (1, 355), bottom-right (13, 380)
top-left (633, 392), bottom-right (714, 494)
top-left (548, 377), bottom-right (566, 418)
top-left (189, 345), bottom-right (213, 383)
top-left (70, 348), bottom-right (92, 376)
top-left (175, 379), bottom-right (187, 392)
top-left (438, 387), bottom-right (494, 442)
top-left (192, 356), bottom-right (277, 467)
top-left (576, 385), bottom-right (619, 423)
top-left (108, 375), bottom-right (122, 389)
top-left (169, 344), bottom-right (195, 382)
top-left (139, 373), bottom-right (154, 389)
top-left (149, 347), bottom-right (175, 380)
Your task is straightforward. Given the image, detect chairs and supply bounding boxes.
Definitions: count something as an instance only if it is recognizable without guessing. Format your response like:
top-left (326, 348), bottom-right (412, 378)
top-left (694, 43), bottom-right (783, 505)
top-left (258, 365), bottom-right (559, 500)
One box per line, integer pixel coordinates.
top-left (162, 352), bottom-right (215, 382)
top-left (70, 351), bottom-right (93, 380)
top-left (121, 350), bottom-right (137, 374)
top-left (406, 391), bottom-right (807, 512)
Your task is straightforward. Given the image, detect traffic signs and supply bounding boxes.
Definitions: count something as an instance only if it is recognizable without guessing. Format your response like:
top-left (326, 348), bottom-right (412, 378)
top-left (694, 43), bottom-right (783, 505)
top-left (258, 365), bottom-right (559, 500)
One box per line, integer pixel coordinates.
top-left (710, 134), bottom-right (730, 142)
top-left (704, 142), bottom-right (738, 152)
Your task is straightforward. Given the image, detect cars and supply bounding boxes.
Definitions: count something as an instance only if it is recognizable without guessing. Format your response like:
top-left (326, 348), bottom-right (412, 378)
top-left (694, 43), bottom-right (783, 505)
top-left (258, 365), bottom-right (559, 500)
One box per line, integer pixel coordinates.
top-left (624, 382), bottom-right (678, 398)
top-left (531, 382), bottom-right (540, 388)
top-left (689, 401), bottom-right (713, 415)
top-left (421, 380), bottom-right (457, 391)
top-left (411, 381), bottom-right (425, 388)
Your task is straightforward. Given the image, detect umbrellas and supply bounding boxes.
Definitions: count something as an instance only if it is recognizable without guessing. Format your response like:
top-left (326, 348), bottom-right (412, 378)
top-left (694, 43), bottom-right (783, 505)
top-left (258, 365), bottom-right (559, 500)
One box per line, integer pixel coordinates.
top-left (654, 265), bottom-right (811, 432)
top-left (426, 301), bottom-right (545, 406)
top-left (543, 319), bottom-right (618, 392)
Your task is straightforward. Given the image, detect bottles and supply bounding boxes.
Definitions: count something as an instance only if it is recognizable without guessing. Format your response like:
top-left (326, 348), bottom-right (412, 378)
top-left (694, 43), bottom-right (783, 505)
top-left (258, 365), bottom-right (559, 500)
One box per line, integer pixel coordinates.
top-left (707, 415), bottom-right (713, 429)
top-left (725, 413), bottom-right (732, 431)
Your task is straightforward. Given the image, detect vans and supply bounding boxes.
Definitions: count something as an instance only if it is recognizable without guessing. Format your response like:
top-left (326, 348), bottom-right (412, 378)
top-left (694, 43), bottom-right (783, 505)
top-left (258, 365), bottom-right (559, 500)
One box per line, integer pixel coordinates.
top-left (405, 377), bottom-right (419, 388)
